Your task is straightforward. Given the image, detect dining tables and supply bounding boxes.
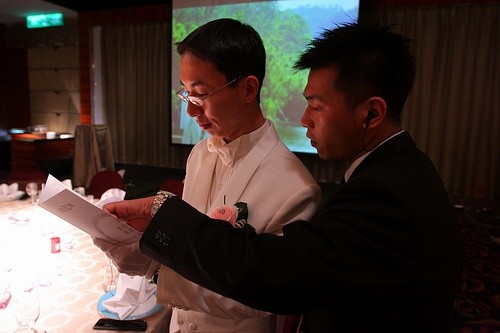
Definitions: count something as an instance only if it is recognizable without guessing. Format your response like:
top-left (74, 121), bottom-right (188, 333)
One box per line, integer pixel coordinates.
top-left (0, 191), bottom-right (172, 333)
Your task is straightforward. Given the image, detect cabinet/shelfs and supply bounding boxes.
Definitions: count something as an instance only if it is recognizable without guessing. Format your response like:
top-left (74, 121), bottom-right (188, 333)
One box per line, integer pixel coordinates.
top-left (9, 134), bottom-right (76, 192)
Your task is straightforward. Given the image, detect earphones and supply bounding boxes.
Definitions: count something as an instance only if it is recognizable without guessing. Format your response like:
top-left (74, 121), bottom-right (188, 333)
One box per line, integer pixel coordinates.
top-left (365, 110), bottom-right (379, 124)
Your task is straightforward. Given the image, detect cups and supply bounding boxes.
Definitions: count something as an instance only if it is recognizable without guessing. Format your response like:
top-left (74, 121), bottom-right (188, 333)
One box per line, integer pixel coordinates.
top-left (31, 124), bottom-right (48, 134)
top-left (50, 237), bottom-right (61, 253)
top-left (46, 131), bottom-right (55, 139)
top-left (74, 186), bottom-right (94, 204)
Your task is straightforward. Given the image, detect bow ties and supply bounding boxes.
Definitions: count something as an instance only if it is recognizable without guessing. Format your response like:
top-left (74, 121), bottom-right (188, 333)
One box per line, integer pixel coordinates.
top-left (207, 126), bottom-right (271, 168)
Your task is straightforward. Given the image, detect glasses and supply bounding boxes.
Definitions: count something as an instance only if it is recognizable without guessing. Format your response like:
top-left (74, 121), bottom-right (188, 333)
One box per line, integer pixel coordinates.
top-left (176, 76), bottom-right (241, 107)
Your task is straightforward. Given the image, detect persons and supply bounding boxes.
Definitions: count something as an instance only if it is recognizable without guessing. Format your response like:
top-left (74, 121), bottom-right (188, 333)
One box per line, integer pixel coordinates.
top-left (102, 21), bottom-right (466, 333)
top-left (94, 18), bottom-right (322, 333)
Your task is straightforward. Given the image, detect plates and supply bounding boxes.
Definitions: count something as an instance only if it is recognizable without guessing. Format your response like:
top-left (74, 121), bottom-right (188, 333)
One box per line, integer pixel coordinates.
top-left (452, 204), bottom-right (500, 333)
top-left (97, 288), bottom-right (163, 319)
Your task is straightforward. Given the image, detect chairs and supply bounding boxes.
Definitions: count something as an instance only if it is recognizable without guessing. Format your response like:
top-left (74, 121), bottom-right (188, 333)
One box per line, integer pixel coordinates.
top-left (88, 171), bottom-right (127, 199)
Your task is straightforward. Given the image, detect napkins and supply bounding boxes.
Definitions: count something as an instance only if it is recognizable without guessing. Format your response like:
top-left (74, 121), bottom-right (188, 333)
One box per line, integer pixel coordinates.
top-left (102, 271), bottom-right (157, 322)
top-left (94, 187), bottom-right (125, 209)
top-left (0, 183), bottom-right (26, 202)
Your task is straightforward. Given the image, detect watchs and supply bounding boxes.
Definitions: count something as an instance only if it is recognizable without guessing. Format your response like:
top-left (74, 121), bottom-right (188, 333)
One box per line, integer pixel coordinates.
top-left (150, 190), bottom-right (177, 218)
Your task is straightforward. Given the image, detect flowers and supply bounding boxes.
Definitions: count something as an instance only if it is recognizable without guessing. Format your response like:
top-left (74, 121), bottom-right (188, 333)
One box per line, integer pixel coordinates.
top-left (211, 204), bottom-right (239, 224)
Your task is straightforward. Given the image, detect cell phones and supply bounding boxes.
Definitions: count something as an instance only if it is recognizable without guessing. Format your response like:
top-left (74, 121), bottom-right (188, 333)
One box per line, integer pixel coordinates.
top-left (94, 317), bottom-right (147, 332)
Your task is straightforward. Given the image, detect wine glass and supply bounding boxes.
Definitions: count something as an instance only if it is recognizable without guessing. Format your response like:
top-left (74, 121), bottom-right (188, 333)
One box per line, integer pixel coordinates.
top-left (26, 182), bottom-right (39, 203)
